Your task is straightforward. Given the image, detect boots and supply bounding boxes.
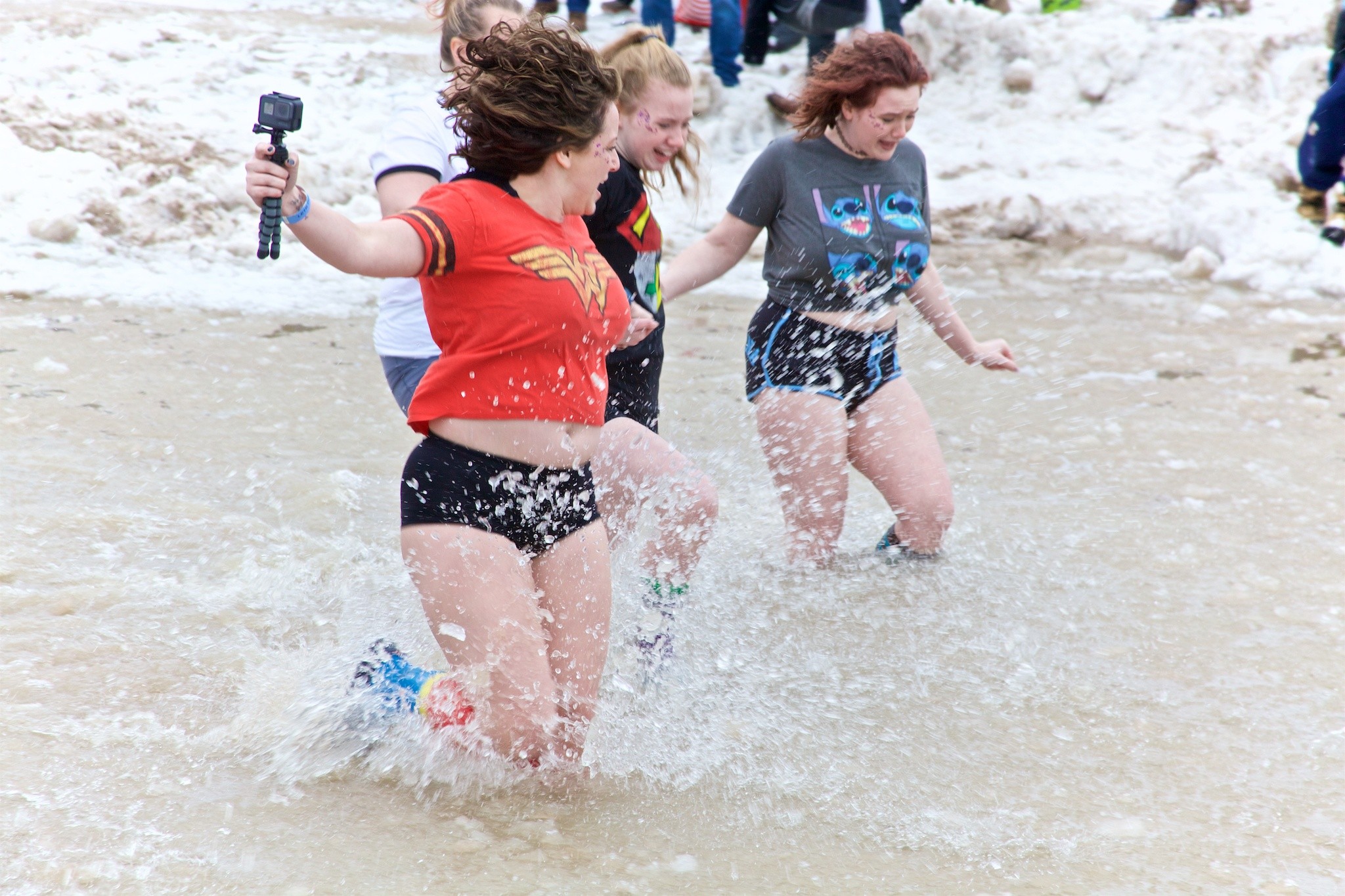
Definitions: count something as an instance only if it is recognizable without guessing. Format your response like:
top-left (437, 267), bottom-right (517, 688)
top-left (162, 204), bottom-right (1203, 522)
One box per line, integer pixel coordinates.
top-left (354, 638), bottom-right (443, 717)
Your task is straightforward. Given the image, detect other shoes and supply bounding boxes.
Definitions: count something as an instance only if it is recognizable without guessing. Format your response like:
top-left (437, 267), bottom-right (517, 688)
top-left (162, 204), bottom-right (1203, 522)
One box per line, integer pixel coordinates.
top-left (1333, 193), bottom-right (1345, 219)
top-left (1296, 184), bottom-right (1330, 223)
top-left (767, 93), bottom-right (804, 115)
top-left (601, 1), bottom-right (635, 16)
top-left (530, 0), bottom-right (558, 16)
top-left (569, 10), bottom-right (587, 32)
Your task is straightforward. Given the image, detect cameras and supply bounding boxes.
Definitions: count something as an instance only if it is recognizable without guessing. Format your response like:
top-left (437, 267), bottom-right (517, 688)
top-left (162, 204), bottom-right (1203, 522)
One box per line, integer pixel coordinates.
top-left (258, 92), bottom-right (303, 131)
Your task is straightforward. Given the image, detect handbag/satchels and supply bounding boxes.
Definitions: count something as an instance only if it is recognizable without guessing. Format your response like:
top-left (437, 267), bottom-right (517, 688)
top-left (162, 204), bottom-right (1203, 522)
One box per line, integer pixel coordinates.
top-left (674, 0), bottom-right (748, 28)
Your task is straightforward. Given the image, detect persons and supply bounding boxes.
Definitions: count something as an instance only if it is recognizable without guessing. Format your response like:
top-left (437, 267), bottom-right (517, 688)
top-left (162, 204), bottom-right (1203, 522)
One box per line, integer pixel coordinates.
top-left (535, 0), bottom-right (589, 32)
top-left (581, 26), bottom-right (719, 658)
top-left (369, 0), bottom-right (527, 419)
top-left (642, 0), bottom-right (904, 87)
top-left (246, 12), bottom-right (632, 766)
top-left (1297, 62), bottom-right (1345, 224)
top-left (659, 30), bottom-right (1017, 567)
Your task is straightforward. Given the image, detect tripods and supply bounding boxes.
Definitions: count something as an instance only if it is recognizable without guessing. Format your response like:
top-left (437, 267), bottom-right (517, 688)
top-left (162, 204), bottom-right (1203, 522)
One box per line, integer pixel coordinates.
top-left (257, 129), bottom-right (289, 260)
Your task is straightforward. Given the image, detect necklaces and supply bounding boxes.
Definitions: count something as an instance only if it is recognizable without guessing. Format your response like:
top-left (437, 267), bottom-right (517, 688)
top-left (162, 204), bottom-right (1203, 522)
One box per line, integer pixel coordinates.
top-left (836, 120), bottom-right (867, 156)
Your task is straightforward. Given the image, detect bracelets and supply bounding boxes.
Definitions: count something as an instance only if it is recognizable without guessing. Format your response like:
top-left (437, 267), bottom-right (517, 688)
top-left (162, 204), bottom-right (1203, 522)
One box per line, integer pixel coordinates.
top-left (281, 184), bottom-right (311, 224)
